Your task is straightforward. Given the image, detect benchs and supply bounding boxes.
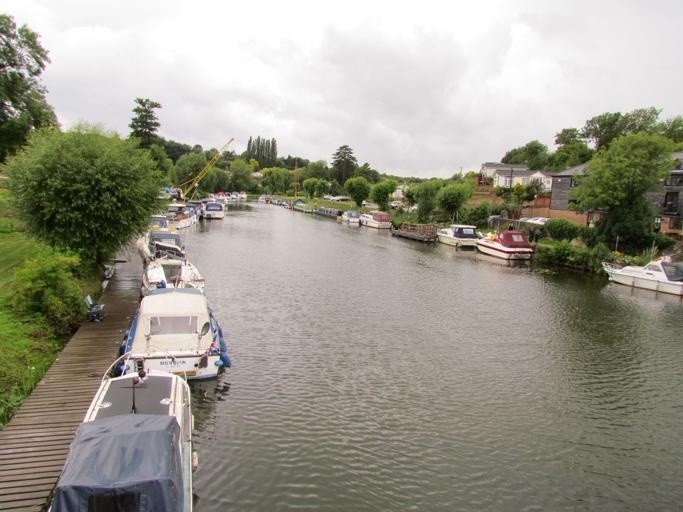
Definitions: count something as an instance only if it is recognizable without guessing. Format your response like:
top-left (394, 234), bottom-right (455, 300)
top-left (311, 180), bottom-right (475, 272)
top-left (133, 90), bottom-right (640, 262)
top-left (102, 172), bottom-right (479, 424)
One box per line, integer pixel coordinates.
top-left (84, 294), bottom-right (106, 323)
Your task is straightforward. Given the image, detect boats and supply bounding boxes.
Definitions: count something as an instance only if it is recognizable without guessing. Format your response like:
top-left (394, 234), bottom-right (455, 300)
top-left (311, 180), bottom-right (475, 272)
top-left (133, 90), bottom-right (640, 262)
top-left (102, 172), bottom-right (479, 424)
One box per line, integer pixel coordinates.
top-left (392, 219), bottom-right (534, 263)
top-left (599, 282), bottom-right (683, 312)
top-left (256, 193), bottom-right (393, 232)
top-left (602, 255), bottom-right (683, 297)
